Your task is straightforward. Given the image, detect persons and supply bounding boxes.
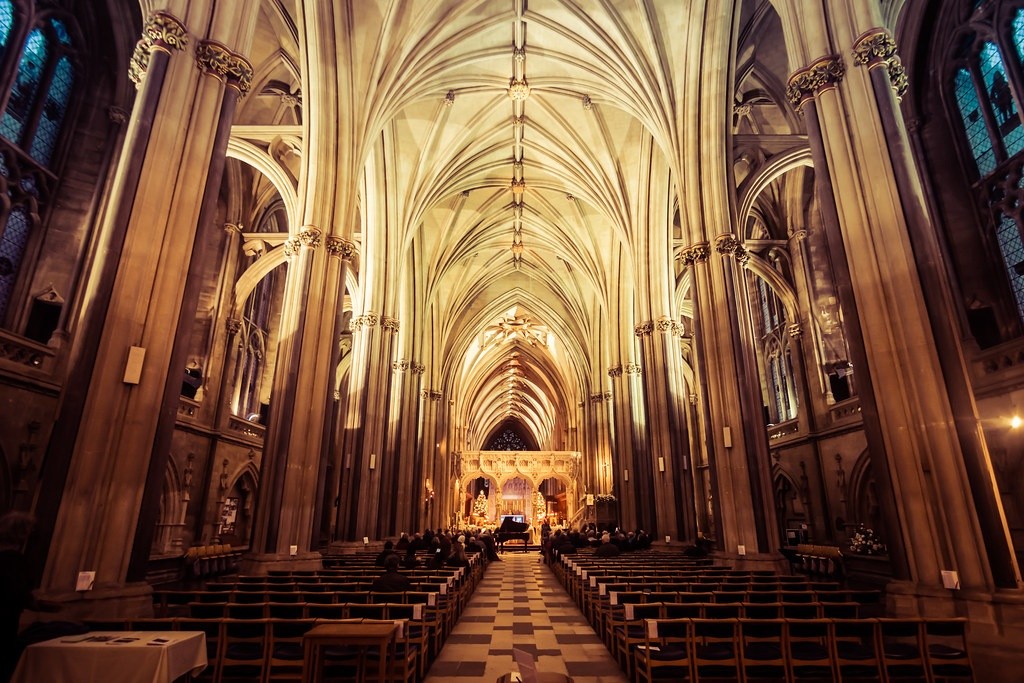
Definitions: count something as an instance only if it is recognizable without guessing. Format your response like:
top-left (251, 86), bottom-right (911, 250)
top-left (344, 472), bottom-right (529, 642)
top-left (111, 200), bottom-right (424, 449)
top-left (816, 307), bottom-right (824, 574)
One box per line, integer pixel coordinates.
top-left (375, 554), bottom-right (413, 603)
top-left (373, 524), bottom-right (500, 576)
top-left (683, 539), bottom-right (707, 556)
top-left (539, 517), bottom-right (652, 568)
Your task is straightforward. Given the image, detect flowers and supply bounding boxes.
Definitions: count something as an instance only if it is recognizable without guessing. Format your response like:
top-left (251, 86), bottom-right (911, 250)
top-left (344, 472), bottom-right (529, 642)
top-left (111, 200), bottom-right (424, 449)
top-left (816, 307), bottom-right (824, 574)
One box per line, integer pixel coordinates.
top-left (850, 523), bottom-right (887, 555)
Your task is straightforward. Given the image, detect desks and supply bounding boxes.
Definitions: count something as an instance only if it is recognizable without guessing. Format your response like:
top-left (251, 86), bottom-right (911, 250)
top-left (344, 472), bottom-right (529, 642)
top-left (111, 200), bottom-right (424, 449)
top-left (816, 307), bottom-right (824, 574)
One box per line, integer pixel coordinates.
top-left (304, 625), bottom-right (399, 683)
top-left (12, 632), bottom-right (207, 683)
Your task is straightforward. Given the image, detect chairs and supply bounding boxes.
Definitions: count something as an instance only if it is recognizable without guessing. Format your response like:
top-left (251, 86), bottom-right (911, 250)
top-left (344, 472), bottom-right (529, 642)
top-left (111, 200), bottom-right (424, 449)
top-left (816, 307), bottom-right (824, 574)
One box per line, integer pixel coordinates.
top-left (81, 543), bottom-right (488, 683)
top-left (543, 543), bottom-right (972, 683)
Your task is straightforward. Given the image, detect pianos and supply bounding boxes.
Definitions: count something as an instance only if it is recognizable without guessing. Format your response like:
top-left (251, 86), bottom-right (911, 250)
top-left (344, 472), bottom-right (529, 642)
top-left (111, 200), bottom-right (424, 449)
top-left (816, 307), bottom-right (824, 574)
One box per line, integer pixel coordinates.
top-left (493, 515), bottom-right (530, 554)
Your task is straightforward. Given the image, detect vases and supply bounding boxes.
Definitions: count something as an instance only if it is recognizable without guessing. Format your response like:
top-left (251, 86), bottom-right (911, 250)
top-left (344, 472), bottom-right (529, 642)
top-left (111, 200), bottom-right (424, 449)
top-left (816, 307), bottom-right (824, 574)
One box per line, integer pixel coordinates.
top-left (847, 554), bottom-right (892, 603)
